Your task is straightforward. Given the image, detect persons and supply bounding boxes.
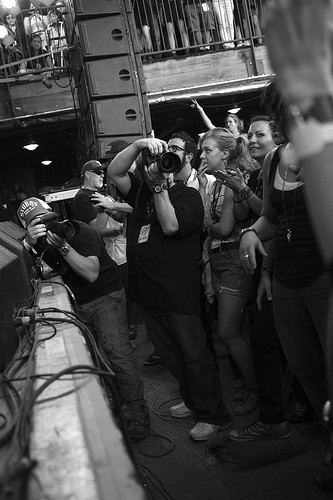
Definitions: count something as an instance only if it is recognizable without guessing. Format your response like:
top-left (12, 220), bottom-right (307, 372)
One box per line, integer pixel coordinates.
top-left (0, 0), bottom-right (333, 487)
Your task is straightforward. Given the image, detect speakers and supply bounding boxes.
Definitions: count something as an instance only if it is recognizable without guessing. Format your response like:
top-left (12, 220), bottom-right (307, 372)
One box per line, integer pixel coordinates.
top-left (0, 220), bottom-right (40, 375)
top-left (64, 0), bottom-right (153, 169)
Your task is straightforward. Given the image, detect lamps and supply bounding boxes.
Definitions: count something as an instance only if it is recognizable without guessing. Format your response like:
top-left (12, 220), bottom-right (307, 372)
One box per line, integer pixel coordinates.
top-left (41, 157), bottom-right (53, 166)
top-left (0, 0), bottom-right (22, 18)
top-left (0, 24), bottom-right (14, 48)
top-left (22, 133), bottom-right (39, 153)
top-left (29, 0), bottom-right (56, 9)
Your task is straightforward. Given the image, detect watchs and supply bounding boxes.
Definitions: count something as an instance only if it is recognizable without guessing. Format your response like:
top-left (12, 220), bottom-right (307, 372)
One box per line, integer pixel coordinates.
top-left (153, 185), bottom-right (167, 193)
top-left (59, 244), bottom-right (72, 255)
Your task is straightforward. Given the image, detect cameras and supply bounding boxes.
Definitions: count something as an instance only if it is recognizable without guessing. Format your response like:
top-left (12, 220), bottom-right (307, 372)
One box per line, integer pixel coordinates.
top-left (154, 151), bottom-right (180, 173)
top-left (45, 219), bottom-right (80, 239)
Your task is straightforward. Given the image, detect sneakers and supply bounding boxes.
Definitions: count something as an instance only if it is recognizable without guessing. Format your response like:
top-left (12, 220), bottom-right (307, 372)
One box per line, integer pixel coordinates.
top-left (189, 419), bottom-right (232, 440)
top-left (289, 399), bottom-right (308, 425)
top-left (226, 418), bottom-right (291, 442)
top-left (169, 402), bottom-right (194, 418)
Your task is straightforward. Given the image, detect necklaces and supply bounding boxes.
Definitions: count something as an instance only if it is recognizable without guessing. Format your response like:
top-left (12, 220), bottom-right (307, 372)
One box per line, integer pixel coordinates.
top-left (282, 143), bottom-right (301, 242)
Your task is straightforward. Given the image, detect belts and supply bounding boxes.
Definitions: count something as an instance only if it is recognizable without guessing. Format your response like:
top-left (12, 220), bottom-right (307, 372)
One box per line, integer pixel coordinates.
top-left (210, 242), bottom-right (240, 254)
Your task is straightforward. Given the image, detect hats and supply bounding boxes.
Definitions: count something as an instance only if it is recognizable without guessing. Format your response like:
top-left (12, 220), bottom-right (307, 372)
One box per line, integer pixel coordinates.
top-left (18, 197), bottom-right (52, 229)
top-left (101, 140), bottom-right (130, 159)
top-left (81, 159), bottom-right (108, 174)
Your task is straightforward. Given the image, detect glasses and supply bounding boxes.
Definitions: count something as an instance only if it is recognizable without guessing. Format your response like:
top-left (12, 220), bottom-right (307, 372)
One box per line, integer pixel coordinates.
top-left (90, 170), bottom-right (107, 176)
top-left (162, 144), bottom-right (190, 155)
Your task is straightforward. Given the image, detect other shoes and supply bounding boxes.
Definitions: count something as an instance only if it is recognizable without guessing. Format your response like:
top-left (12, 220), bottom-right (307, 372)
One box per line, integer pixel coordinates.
top-left (231, 379), bottom-right (245, 386)
top-left (127, 415), bottom-right (151, 438)
top-left (129, 325), bottom-right (138, 339)
top-left (143, 38), bottom-right (261, 62)
top-left (230, 391), bottom-right (261, 416)
top-left (233, 389), bottom-right (250, 402)
top-left (142, 354), bottom-right (161, 364)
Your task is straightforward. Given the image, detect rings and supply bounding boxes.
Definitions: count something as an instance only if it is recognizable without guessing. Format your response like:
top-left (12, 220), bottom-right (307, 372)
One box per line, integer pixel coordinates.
top-left (243, 255), bottom-right (249, 259)
top-left (197, 173), bottom-right (200, 175)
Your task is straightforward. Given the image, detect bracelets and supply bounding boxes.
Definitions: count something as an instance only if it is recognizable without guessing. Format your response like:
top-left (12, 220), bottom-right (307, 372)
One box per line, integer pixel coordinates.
top-left (232, 198), bottom-right (244, 203)
top-left (240, 227), bottom-right (258, 238)
top-left (23, 240), bottom-right (37, 254)
top-left (262, 267), bottom-right (273, 274)
top-left (240, 187), bottom-right (254, 199)
